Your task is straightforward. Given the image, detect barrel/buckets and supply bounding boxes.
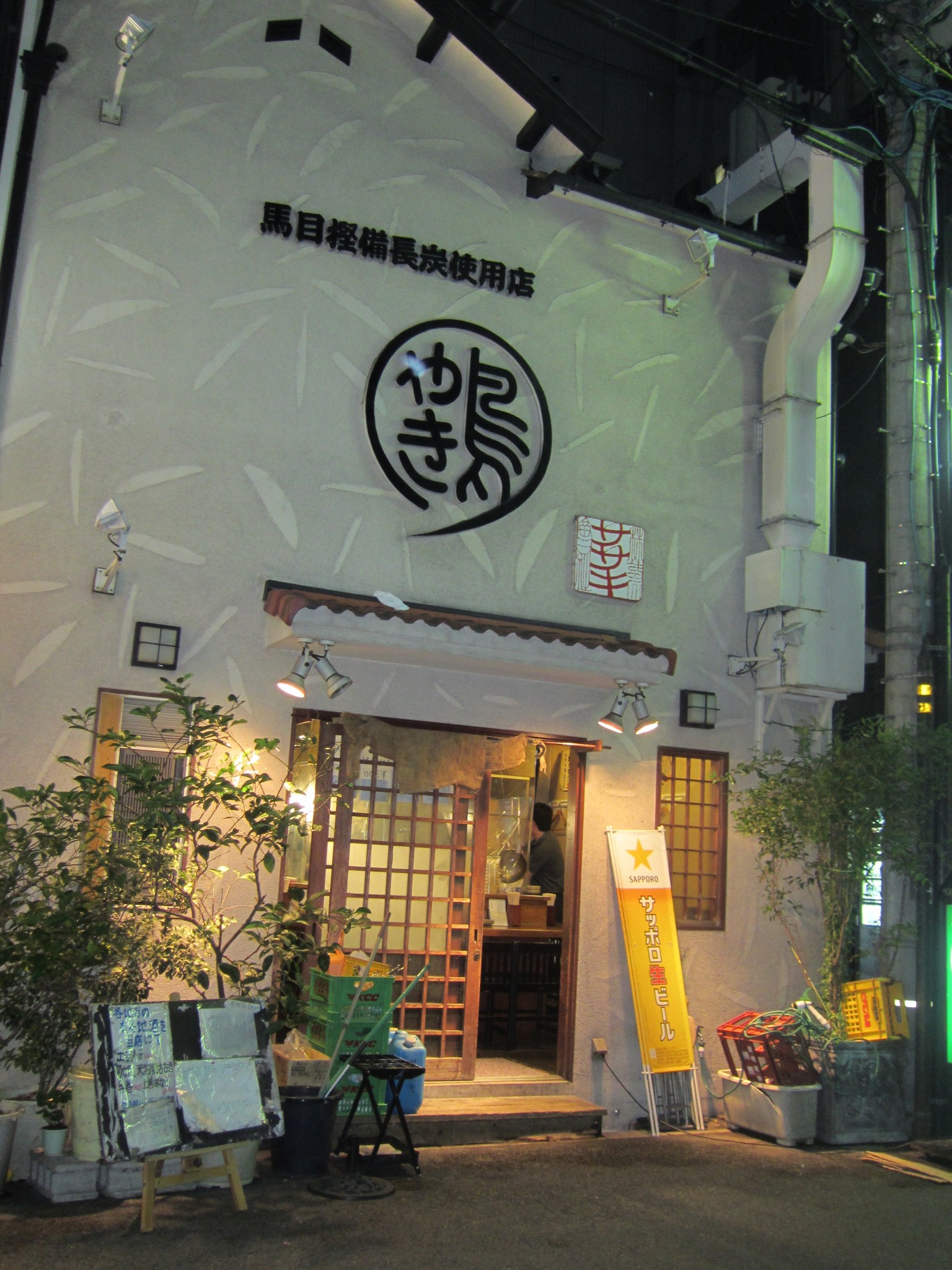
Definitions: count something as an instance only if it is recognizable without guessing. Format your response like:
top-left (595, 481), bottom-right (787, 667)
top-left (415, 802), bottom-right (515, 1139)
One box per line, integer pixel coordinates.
top-left (384, 1027), bottom-right (427, 1113)
top-left (269, 1085), bottom-right (344, 1179)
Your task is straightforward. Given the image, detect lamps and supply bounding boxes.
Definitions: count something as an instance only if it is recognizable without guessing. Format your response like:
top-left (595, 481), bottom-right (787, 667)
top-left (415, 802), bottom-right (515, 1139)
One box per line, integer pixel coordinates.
top-left (89, 497), bottom-right (132, 600)
top-left (276, 637), bottom-right (354, 700)
top-left (595, 678), bottom-right (660, 737)
top-left (98, 14), bottom-right (159, 127)
top-left (659, 226), bottom-right (720, 317)
top-left (726, 621), bottom-right (807, 678)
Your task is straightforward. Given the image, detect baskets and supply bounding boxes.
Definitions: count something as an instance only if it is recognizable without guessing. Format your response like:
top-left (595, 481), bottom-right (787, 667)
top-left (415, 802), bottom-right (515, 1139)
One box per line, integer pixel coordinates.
top-left (330, 1067), bottom-right (387, 1115)
top-left (716, 1010), bottom-right (818, 1086)
top-left (308, 968), bottom-right (395, 1022)
top-left (295, 1013), bottom-right (391, 1068)
top-left (841, 977), bottom-right (910, 1041)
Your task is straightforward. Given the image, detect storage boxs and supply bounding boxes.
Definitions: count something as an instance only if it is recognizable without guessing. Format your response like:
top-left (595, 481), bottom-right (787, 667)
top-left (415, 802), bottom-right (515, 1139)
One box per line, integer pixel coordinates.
top-left (715, 1008), bottom-right (816, 1086)
top-left (836, 977), bottom-right (912, 1044)
top-left (302, 948), bottom-right (396, 1118)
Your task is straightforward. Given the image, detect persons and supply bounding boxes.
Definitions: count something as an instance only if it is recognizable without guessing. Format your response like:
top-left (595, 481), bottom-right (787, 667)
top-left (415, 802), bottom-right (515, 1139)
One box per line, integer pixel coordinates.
top-left (522, 802), bottom-right (565, 922)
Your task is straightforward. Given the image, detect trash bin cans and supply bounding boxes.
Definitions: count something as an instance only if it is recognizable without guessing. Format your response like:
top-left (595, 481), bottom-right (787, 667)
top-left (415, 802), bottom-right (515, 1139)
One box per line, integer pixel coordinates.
top-left (273, 1086), bottom-right (345, 1178)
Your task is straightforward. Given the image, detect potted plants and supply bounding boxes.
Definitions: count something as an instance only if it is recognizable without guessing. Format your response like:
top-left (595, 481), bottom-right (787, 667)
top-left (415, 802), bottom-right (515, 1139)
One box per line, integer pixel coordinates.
top-left (0, 771), bottom-right (239, 1192)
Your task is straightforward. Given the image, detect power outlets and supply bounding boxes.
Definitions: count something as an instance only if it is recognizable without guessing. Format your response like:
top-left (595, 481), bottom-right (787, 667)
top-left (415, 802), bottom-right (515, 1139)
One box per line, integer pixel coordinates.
top-left (592, 1037), bottom-right (608, 1059)
